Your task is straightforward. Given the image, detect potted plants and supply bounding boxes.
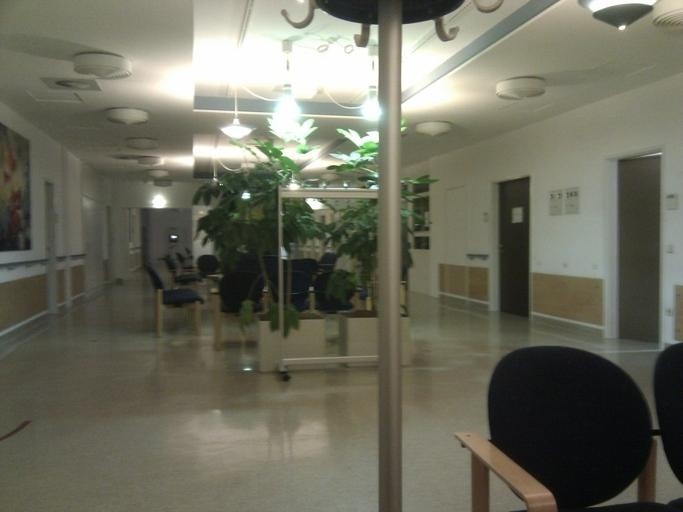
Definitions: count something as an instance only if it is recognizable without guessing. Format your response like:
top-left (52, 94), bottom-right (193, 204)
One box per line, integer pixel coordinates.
top-left (191, 117), bottom-right (336, 373)
top-left (314, 118), bottom-right (440, 368)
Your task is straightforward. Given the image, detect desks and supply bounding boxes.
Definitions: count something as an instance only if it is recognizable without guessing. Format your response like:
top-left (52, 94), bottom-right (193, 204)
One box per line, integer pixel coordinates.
top-left (370, 280), bottom-right (407, 315)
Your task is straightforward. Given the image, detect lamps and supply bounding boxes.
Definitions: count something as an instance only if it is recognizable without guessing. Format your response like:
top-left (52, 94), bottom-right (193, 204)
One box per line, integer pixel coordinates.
top-left (577, 0), bottom-right (662, 33)
top-left (219, 84), bottom-right (253, 139)
top-left (415, 122), bottom-right (453, 138)
top-left (71, 53), bottom-right (133, 81)
top-left (123, 138), bottom-right (173, 187)
top-left (104, 107), bottom-right (150, 127)
top-left (496, 77), bottom-right (545, 100)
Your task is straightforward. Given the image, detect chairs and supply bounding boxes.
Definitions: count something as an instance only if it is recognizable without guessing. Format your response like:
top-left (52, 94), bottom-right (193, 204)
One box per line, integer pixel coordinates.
top-left (456, 345), bottom-right (673, 511)
top-left (142, 246), bottom-right (375, 342)
top-left (654, 341), bottom-right (683, 512)
